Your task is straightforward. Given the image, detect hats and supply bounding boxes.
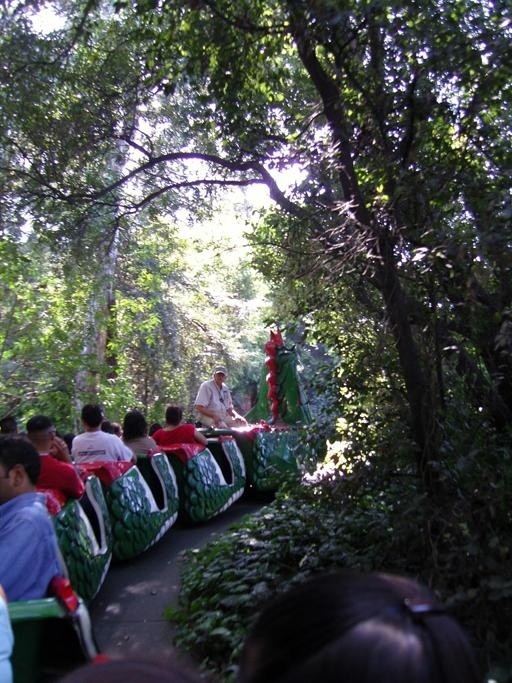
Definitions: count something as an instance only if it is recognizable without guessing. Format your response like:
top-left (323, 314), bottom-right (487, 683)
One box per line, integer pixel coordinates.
top-left (215, 366), bottom-right (227, 377)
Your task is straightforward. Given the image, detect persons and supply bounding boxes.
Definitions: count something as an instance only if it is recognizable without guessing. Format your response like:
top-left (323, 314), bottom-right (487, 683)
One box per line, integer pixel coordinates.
top-left (233, 564), bottom-right (479, 681)
top-left (194, 365), bottom-right (247, 429)
